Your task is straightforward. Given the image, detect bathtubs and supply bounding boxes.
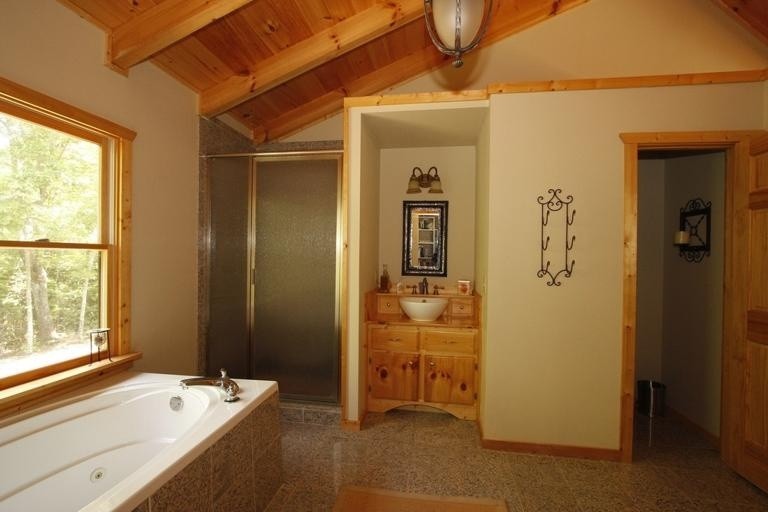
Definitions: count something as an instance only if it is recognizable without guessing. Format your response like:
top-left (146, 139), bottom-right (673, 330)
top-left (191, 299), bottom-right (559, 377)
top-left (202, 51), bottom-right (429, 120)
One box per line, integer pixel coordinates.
top-left (0, 372), bottom-right (279, 511)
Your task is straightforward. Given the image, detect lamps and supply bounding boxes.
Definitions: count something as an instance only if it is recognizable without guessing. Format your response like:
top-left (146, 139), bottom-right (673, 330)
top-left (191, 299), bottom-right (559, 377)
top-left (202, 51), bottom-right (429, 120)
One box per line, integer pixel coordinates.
top-left (672, 198), bottom-right (713, 264)
top-left (407, 163), bottom-right (444, 194)
top-left (423, 0), bottom-right (492, 68)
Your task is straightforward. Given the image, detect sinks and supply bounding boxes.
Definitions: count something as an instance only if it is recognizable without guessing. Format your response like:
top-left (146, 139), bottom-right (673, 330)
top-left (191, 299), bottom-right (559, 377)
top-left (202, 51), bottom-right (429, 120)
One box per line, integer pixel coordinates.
top-left (398, 297), bottom-right (448, 322)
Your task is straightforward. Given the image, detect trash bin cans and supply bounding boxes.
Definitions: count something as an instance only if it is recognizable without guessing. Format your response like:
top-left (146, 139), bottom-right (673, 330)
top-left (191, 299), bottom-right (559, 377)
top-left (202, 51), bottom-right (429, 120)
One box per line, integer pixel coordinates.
top-left (638, 380), bottom-right (666, 417)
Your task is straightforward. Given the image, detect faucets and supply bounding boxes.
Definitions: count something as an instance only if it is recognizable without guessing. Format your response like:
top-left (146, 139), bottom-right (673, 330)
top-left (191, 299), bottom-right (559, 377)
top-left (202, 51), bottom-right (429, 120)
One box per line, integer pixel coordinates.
top-left (422, 277), bottom-right (429, 293)
top-left (176, 366), bottom-right (240, 402)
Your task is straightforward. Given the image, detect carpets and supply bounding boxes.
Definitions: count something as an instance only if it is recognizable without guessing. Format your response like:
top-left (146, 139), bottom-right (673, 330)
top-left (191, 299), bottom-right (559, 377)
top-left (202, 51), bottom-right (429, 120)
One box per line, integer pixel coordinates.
top-left (334, 480), bottom-right (508, 511)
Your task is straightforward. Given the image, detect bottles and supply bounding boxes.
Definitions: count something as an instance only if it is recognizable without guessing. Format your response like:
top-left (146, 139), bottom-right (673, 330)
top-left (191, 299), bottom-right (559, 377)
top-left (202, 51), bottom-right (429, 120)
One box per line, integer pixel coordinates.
top-left (379, 264), bottom-right (392, 293)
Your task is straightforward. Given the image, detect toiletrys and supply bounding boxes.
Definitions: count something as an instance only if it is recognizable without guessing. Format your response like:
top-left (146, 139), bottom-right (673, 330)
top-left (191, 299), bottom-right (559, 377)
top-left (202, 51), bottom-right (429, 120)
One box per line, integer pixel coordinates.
top-left (397, 280), bottom-right (404, 293)
top-left (379, 265), bottom-right (391, 292)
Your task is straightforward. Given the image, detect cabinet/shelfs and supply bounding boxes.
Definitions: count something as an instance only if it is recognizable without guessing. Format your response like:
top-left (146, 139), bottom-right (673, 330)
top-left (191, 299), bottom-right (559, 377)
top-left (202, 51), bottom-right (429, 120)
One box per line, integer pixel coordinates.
top-left (368, 326), bottom-right (479, 405)
top-left (416, 212), bottom-right (440, 267)
top-left (448, 298), bottom-right (474, 317)
top-left (377, 295), bottom-right (401, 314)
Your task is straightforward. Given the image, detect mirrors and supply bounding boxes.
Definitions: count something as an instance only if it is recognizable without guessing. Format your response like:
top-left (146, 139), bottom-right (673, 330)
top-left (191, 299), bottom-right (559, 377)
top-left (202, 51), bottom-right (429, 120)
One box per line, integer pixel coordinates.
top-left (403, 199), bottom-right (447, 277)
